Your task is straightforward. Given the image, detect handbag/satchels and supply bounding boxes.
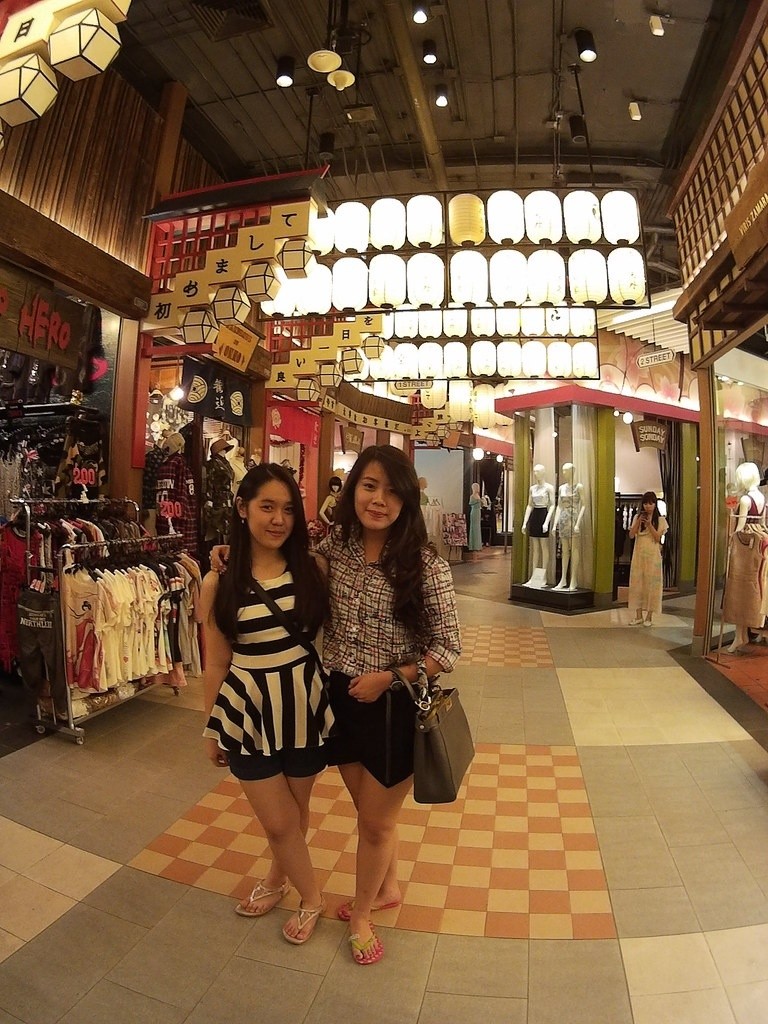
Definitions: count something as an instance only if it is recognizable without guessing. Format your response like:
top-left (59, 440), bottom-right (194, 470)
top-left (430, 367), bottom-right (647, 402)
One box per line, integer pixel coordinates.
top-left (383, 655), bottom-right (475, 804)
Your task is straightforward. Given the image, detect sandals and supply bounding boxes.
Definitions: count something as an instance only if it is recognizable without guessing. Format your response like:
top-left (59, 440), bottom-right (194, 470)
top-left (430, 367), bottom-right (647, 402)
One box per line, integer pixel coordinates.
top-left (281, 890), bottom-right (328, 945)
top-left (234, 878), bottom-right (291, 916)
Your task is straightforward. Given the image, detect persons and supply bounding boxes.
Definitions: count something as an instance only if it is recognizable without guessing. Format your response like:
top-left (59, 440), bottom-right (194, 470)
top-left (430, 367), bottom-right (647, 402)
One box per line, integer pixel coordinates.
top-left (723, 462), bottom-right (766, 652)
top-left (550, 463), bottom-right (585, 591)
top-left (468, 483), bottom-right (483, 552)
top-left (210, 444), bottom-right (461, 964)
top-left (417, 476), bottom-right (433, 544)
top-left (628, 491), bottom-right (670, 628)
top-left (520, 465), bottom-right (556, 588)
top-left (200, 462), bottom-right (338, 945)
top-left (141, 433), bottom-right (260, 580)
top-left (320, 477), bottom-right (343, 536)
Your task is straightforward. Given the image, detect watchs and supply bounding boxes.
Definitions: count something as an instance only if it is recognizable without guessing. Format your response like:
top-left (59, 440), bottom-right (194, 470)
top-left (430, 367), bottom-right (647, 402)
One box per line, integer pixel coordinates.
top-left (389, 672), bottom-right (404, 692)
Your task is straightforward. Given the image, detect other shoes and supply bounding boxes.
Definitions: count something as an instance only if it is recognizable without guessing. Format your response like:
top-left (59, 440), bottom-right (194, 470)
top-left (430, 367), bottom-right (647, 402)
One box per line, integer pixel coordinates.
top-left (643, 615), bottom-right (653, 626)
top-left (628, 617), bottom-right (644, 625)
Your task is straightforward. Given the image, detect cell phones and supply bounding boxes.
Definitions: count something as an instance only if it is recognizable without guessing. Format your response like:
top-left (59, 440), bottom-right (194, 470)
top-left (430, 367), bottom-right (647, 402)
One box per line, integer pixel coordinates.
top-left (640, 511), bottom-right (648, 519)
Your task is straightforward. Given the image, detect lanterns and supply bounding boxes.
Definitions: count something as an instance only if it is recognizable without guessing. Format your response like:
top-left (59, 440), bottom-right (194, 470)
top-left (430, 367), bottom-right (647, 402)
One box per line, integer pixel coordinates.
top-left (260, 189), bottom-right (646, 430)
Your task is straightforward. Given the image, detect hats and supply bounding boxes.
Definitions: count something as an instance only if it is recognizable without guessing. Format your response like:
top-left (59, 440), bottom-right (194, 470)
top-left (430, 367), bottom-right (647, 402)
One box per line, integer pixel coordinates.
top-left (159, 431), bottom-right (186, 463)
top-left (210, 439), bottom-right (234, 459)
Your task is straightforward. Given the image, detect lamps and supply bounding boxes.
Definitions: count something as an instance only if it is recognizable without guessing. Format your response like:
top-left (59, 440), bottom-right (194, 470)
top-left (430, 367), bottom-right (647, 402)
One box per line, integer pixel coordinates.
top-left (575, 29), bottom-right (597, 63)
top-left (448, 193), bottom-right (486, 247)
top-left (422, 39), bottom-right (438, 65)
top-left (394, 343), bottom-right (418, 379)
top-left (494, 383), bottom-right (515, 426)
top-left (522, 340), bottom-right (547, 378)
top-left (406, 195), bottom-right (442, 248)
top-left (343, 347), bottom-right (370, 381)
top-left (495, 308), bottom-right (521, 337)
top-left (417, 341), bottom-right (443, 379)
top-left (547, 342), bottom-right (572, 378)
top-left (444, 342), bottom-right (468, 378)
top-left (435, 84), bottom-right (449, 108)
top-left (369, 253), bottom-right (406, 309)
top-left (524, 191), bottom-right (563, 246)
top-left (527, 249), bottom-right (566, 308)
top-left (490, 249), bottom-right (527, 306)
top-left (275, 55), bottom-right (294, 87)
top-left (569, 308), bottom-right (595, 337)
top-left (545, 308), bottom-right (570, 337)
top-left (394, 302), bottom-right (419, 339)
top-left (563, 190), bottom-right (602, 245)
top-left (570, 115), bottom-right (586, 144)
top-left (374, 312), bottom-right (394, 340)
top-left (450, 250), bottom-right (489, 309)
top-left (312, 206), bottom-right (335, 257)
top-left (420, 380), bottom-right (447, 410)
top-left (419, 305), bottom-right (443, 339)
top-left (470, 340), bottom-right (496, 377)
top-left (407, 252), bottom-right (444, 309)
top-left (497, 341), bottom-right (522, 378)
top-left (601, 190), bottom-right (639, 245)
top-left (470, 309), bottom-right (496, 337)
top-left (449, 380), bottom-right (473, 422)
top-left (412, 0), bottom-right (427, 24)
top-left (442, 302), bottom-right (467, 337)
top-left (649, 16), bottom-right (664, 37)
top-left (486, 189), bottom-right (525, 244)
top-left (568, 249), bottom-right (608, 307)
top-left (295, 263), bottom-right (332, 315)
top-left (391, 382), bottom-right (418, 398)
top-left (607, 245), bottom-right (647, 306)
top-left (260, 278), bottom-right (295, 317)
top-left (572, 342), bottom-right (597, 377)
top-left (473, 384), bottom-right (496, 430)
top-left (346, 317), bottom-right (373, 342)
top-left (318, 132), bottom-right (334, 160)
top-left (370, 198), bottom-right (407, 251)
top-left (370, 345), bottom-right (394, 380)
top-left (521, 308), bottom-right (545, 336)
top-left (332, 257), bottom-right (369, 311)
top-left (335, 201), bottom-right (370, 254)
top-left (627, 102), bottom-right (641, 121)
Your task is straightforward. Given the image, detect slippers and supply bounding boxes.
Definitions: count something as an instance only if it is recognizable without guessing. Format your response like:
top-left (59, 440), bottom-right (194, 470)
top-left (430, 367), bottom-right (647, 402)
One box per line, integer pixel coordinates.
top-left (347, 920), bottom-right (385, 966)
top-left (336, 898), bottom-right (400, 921)
